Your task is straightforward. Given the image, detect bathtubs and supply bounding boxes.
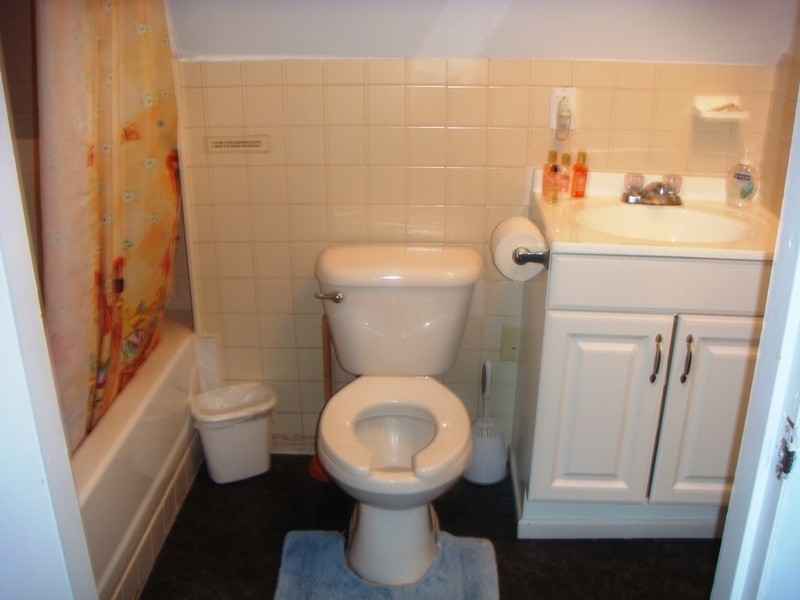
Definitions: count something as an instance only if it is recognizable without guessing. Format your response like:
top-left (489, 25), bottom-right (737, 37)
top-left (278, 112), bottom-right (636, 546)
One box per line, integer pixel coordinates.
top-left (62, 312), bottom-right (193, 587)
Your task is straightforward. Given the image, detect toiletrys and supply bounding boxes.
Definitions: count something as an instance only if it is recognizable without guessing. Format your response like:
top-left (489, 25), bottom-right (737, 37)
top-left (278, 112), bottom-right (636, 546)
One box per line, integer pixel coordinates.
top-left (541, 148), bottom-right (588, 208)
top-left (727, 145), bottom-right (759, 210)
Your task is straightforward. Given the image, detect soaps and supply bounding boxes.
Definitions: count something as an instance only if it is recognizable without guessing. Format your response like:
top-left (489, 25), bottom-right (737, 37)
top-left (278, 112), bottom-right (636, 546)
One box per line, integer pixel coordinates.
top-left (705, 104), bottom-right (742, 114)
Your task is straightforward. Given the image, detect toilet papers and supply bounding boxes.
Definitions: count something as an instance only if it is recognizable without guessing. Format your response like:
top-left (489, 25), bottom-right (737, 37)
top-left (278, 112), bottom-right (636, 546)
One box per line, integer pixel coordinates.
top-left (489, 216), bottom-right (548, 280)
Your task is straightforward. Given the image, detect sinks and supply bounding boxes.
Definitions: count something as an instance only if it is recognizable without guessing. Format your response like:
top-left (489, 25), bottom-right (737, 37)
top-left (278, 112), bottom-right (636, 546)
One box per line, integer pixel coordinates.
top-left (565, 204), bottom-right (751, 247)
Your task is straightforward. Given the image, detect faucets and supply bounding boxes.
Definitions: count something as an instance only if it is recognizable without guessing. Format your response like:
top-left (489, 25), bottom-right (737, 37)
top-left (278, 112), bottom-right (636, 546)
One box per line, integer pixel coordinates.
top-left (623, 172), bottom-right (684, 206)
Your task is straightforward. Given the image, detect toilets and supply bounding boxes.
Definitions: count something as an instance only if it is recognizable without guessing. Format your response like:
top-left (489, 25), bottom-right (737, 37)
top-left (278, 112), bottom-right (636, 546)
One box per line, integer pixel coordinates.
top-left (312, 242), bottom-right (484, 586)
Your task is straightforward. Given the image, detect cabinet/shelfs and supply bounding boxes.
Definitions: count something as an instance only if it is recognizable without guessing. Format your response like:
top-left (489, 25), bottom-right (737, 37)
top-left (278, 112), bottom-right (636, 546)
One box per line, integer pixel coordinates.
top-left (511, 257), bottom-right (774, 540)
